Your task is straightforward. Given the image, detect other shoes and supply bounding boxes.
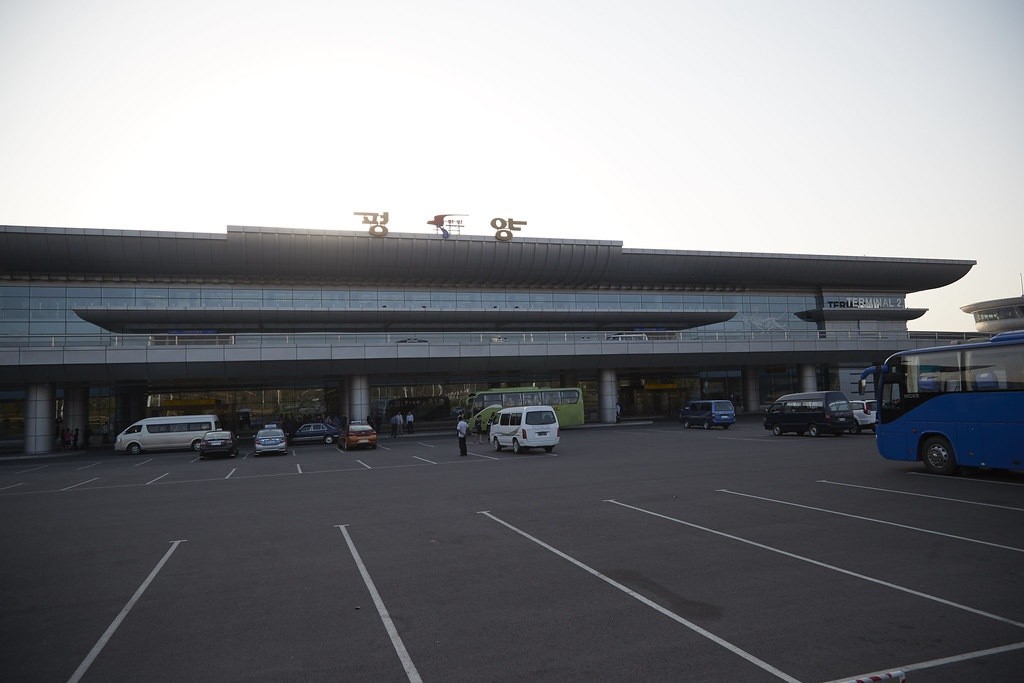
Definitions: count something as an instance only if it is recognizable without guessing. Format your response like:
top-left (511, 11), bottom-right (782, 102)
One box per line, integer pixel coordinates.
top-left (459, 453), bottom-right (467, 456)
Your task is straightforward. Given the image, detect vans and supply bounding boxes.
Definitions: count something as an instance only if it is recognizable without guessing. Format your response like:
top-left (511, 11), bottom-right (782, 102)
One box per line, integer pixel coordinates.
top-left (489, 405), bottom-right (560, 454)
top-left (827, 399), bottom-right (877, 434)
top-left (115, 414), bottom-right (222, 455)
top-left (763, 390), bottom-right (856, 438)
top-left (678, 399), bottom-right (736, 430)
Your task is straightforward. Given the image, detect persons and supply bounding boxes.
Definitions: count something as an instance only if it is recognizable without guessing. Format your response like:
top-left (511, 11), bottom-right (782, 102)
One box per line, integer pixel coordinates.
top-left (457, 416), bottom-right (469, 456)
top-left (473, 415), bottom-right (486, 444)
top-left (102, 421), bottom-right (110, 444)
top-left (366, 410), bottom-right (415, 438)
top-left (615, 403), bottom-right (621, 424)
top-left (276, 411), bottom-right (348, 435)
top-left (486, 411), bottom-right (496, 441)
top-left (55, 423), bottom-right (93, 451)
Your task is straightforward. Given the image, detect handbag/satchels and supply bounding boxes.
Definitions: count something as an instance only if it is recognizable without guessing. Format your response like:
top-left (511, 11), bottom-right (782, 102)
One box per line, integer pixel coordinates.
top-left (472, 428), bottom-right (477, 433)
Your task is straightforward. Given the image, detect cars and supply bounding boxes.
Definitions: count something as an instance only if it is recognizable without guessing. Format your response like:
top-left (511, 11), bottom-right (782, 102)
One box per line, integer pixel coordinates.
top-left (287, 422), bottom-right (342, 444)
top-left (199, 428), bottom-right (240, 459)
top-left (336, 421), bottom-right (378, 452)
top-left (252, 424), bottom-right (289, 457)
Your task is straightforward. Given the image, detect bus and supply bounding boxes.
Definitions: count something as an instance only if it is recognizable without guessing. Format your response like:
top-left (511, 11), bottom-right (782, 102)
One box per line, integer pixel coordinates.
top-left (856, 329), bottom-right (1024, 483)
top-left (384, 395), bottom-right (451, 422)
top-left (458, 386), bottom-right (585, 435)
